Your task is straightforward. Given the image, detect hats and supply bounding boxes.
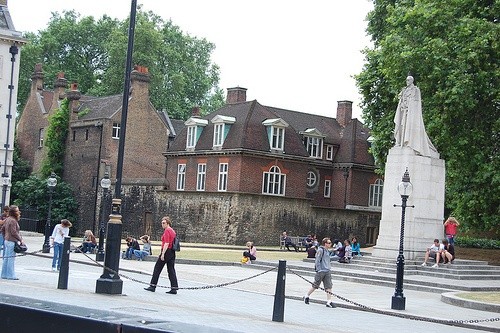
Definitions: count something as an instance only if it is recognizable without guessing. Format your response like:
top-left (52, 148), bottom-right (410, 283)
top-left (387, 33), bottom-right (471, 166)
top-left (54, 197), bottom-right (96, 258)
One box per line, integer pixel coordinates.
top-left (247, 241), bottom-right (253, 247)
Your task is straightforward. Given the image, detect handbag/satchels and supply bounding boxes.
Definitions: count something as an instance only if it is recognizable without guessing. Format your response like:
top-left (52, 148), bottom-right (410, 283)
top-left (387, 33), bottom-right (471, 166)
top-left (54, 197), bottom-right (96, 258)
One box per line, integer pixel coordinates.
top-left (75, 245), bottom-right (85, 252)
top-left (14, 245), bottom-right (28, 253)
top-left (241, 257), bottom-right (248, 264)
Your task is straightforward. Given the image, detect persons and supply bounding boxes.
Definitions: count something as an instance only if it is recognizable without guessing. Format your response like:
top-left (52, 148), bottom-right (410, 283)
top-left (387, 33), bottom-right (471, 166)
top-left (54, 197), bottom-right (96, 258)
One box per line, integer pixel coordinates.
top-left (303, 238), bottom-right (337, 308)
top-left (281, 232), bottom-right (360, 260)
top-left (0, 206), bottom-right (9, 246)
top-left (394, 75), bottom-right (430, 155)
top-left (134, 235), bottom-right (150, 261)
top-left (243, 242), bottom-right (256, 260)
top-left (421, 216), bottom-right (460, 268)
top-left (0, 205), bottom-right (25, 280)
top-left (82, 230), bottom-right (97, 253)
top-left (124, 238), bottom-right (140, 259)
top-left (51, 220), bottom-right (72, 270)
top-left (144, 217), bottom-right (178, 294)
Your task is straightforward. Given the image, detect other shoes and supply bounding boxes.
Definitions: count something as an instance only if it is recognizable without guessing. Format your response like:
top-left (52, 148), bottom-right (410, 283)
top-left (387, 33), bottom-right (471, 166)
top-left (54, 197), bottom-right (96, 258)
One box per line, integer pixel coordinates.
top-left (431, 264), bottom-right (438, 268)
top-left (144, 287), bottom-right (155, 292)
top-left (246, 261), bottom-right (251, 264)
top-left (303, 296), bottom-right (310, 304)
top-left (325, 302), bottom-right (337, 308)
top-left (166, 289), bottom-right (177, 294)
top-left (421, 262), bottom-right (428, 266)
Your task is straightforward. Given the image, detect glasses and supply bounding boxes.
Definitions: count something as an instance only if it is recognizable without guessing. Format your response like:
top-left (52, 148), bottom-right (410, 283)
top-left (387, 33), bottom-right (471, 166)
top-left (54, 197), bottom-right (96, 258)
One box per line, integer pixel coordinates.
top-left (326, 241), bottom-right (331, 244)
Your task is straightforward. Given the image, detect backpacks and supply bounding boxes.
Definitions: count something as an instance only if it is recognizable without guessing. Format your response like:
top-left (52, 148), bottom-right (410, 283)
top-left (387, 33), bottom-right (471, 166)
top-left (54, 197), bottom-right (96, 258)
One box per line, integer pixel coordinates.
top-left (314, 247), bottom-right (324, 271)
top-left (172, 236), bottom-right (181, 251)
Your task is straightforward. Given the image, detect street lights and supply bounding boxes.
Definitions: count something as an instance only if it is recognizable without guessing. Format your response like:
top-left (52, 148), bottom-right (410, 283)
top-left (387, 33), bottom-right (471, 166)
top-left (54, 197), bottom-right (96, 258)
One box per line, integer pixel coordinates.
top-left (390, 167), bottom-right (415, 311)
top-left (343, 169), bottom-right (350, 209)
top-left (42, 171), bottom-right (58, 253)
top-left (95, 169), bottom-right (112, 262)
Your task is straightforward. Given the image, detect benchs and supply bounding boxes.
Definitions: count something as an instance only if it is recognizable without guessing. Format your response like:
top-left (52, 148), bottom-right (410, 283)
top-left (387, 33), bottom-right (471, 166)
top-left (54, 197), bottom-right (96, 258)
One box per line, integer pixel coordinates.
top-left (279, 235), bottom-right (299, 251)
top-left (299, 236), bottom-right (313, 251)
